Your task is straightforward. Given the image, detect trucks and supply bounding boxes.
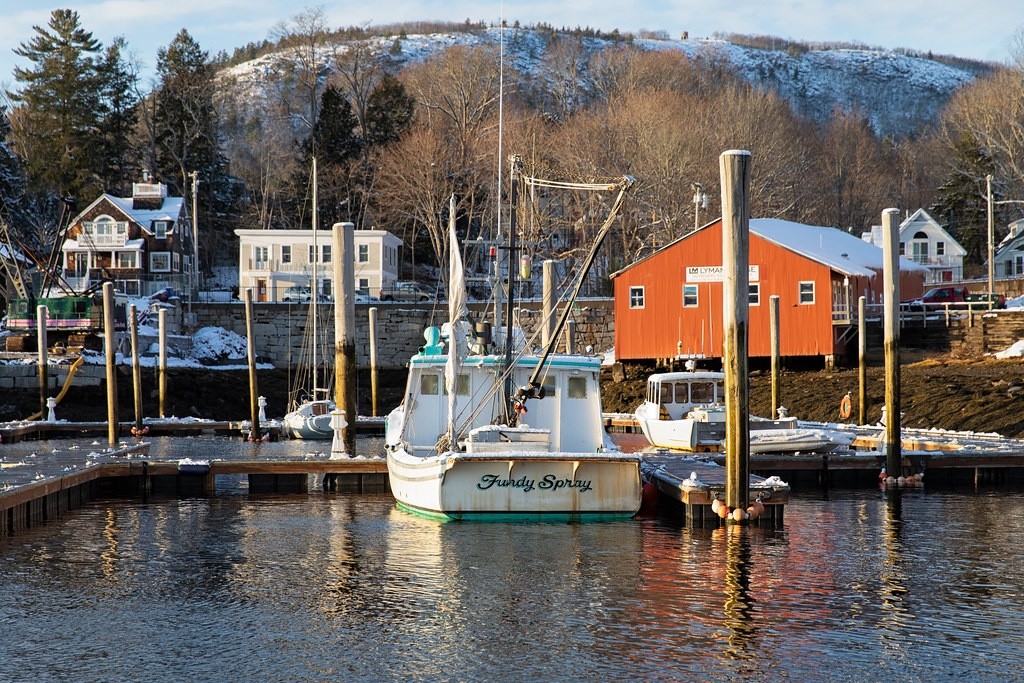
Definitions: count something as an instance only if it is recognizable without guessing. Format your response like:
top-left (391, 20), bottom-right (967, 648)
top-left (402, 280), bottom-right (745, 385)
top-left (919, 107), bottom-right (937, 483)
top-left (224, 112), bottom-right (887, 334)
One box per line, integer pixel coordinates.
top-left (900, 287), bottom-right (1006, 312)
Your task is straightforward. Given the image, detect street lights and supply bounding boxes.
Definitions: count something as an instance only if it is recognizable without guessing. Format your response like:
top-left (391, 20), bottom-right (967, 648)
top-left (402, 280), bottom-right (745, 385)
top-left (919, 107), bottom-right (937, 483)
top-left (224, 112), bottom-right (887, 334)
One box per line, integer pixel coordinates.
top-left (974, 173), bottom-right (996, 313)
top-left (186, 169), bottom-right (202, 299)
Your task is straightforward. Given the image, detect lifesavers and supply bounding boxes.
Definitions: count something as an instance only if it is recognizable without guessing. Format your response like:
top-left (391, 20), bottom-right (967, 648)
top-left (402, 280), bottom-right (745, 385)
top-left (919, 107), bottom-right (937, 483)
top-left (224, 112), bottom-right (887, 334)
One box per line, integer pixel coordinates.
top-left (840, 395), bottom-right (851, 418)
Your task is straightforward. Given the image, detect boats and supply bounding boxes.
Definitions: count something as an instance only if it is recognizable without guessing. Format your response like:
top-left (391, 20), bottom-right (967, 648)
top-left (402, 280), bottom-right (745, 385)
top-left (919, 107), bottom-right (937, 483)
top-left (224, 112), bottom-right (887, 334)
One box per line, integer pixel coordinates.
top-left (376, 14), bottom-right (647, 528)
top-left (596, 367), bottom-right (1010, 456)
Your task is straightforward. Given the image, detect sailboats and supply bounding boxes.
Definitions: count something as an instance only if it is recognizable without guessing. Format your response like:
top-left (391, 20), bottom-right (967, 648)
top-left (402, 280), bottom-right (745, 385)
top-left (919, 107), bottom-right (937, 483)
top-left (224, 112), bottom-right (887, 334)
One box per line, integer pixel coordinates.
top-left (278, 156), bottom-right (339, 441)
